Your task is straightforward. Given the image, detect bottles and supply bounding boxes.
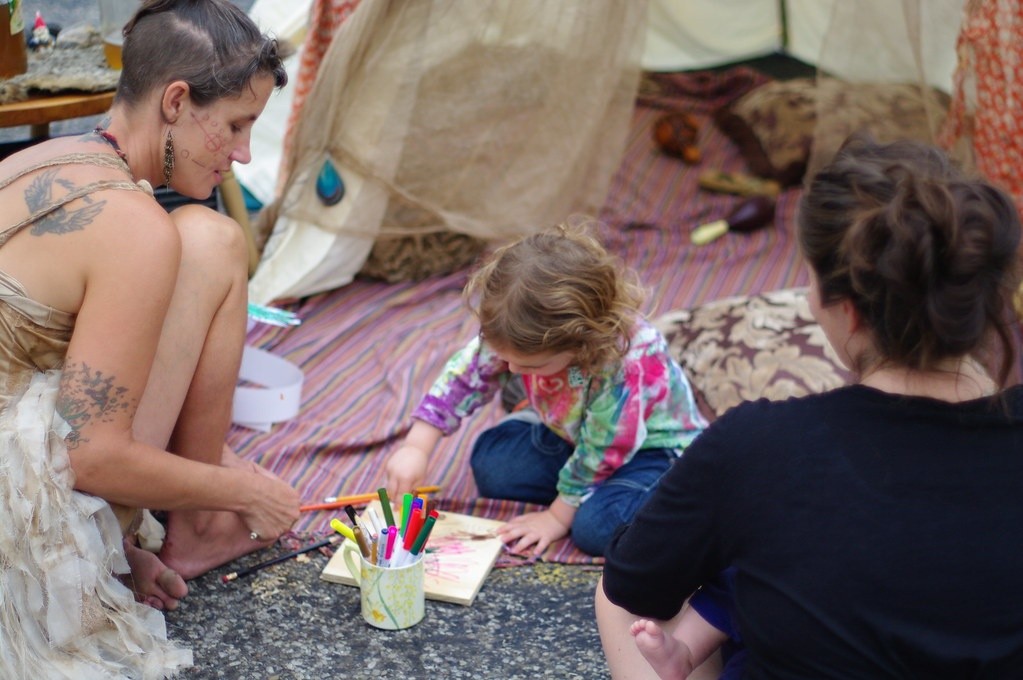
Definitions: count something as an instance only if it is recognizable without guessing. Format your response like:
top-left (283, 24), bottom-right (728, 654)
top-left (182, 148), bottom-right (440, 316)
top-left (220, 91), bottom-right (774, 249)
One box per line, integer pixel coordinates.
top-left (0, 0), bottom-right (28, 81)
top-left (29, 10), bottom-right (56, 55)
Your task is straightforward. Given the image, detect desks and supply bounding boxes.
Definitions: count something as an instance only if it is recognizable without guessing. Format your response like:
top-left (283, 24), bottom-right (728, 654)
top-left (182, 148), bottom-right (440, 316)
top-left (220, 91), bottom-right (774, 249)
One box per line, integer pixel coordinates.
top-left (0, 87), bottom-right (261, 277)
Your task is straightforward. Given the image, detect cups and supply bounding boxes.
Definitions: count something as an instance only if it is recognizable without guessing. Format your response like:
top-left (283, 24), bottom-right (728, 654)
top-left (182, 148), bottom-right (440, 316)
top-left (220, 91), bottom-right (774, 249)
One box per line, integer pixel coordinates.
top-left (343, 542), bottom-right (426, 630)
top-left (101, 26), bottom-right (125, 71)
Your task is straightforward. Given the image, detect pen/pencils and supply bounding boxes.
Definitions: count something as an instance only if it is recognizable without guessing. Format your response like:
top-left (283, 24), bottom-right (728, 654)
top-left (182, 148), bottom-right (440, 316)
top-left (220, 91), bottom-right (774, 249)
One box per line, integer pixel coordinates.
top-left (324, 485), bottom-right (439, 504)
top-left (330, 488), bottom-right (439, 567)
top-left (299, 498), bottom-right (369, 513)
top-left (221, 534), bottom-right (340, 583)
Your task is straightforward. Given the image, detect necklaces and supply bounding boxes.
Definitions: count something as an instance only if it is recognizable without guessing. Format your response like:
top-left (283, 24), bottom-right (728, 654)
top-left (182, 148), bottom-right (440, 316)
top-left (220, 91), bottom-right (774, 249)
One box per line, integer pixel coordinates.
top-left (94, 126), bottom-right (128, 164)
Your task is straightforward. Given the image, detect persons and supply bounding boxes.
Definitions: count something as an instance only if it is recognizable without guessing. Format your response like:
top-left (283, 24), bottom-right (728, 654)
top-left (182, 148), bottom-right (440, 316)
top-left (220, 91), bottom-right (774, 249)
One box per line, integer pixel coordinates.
top-left (386, 226), bottom-right (709, 555)
top-left (0, 0), bottom-right (301, 611)
top-left (595, 129), bottom-right (1023, 680)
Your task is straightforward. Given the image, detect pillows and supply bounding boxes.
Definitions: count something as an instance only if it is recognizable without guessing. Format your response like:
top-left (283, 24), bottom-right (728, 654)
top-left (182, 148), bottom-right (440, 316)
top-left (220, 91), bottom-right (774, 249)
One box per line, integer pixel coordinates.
top-left (713, 80), bottom-right (976, 183)
top-left (651, 286), bottom-right (859, 425)
